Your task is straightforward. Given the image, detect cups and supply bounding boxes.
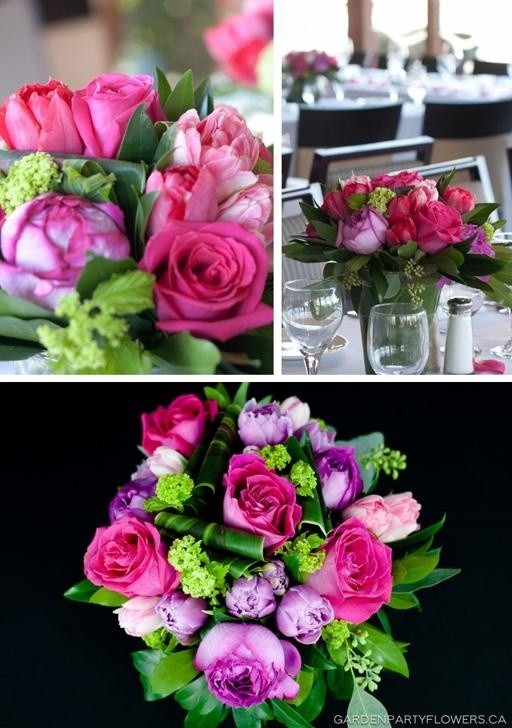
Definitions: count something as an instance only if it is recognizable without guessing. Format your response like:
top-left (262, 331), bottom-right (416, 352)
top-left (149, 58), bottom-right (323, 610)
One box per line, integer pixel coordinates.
top-left (366, 302), bottom-right (430, 375)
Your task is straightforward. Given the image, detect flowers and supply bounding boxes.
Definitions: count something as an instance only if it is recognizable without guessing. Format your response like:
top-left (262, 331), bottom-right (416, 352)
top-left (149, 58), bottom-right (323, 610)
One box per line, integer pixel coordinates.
top-left (293, 163), bottom-right (509, 352)
top-left (63, 382), bottom-right (464, 727)
top-left (0, 68), bottom-right (276, 376)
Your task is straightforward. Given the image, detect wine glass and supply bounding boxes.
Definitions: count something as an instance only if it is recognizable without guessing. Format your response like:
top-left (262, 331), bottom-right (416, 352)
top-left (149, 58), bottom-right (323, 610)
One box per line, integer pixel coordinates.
top-left (281, 280), bottom-right (343, 375)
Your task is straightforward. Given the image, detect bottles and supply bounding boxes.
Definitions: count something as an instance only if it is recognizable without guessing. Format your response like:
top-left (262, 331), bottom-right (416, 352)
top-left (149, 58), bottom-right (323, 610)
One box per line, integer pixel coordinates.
top-left (442, 297), bottom-right (478, 376)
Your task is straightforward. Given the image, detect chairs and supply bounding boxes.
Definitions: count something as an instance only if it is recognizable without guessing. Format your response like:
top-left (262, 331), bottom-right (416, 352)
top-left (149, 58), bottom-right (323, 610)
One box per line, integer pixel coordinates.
top-left (307, 136), bottom-right (434, 188)
top-left (287, 99), bottom-right (403, 183)
top-left (422, 96), bottom-right (507, 224)
top-left (383, 153), bottom-right (506, 244)
